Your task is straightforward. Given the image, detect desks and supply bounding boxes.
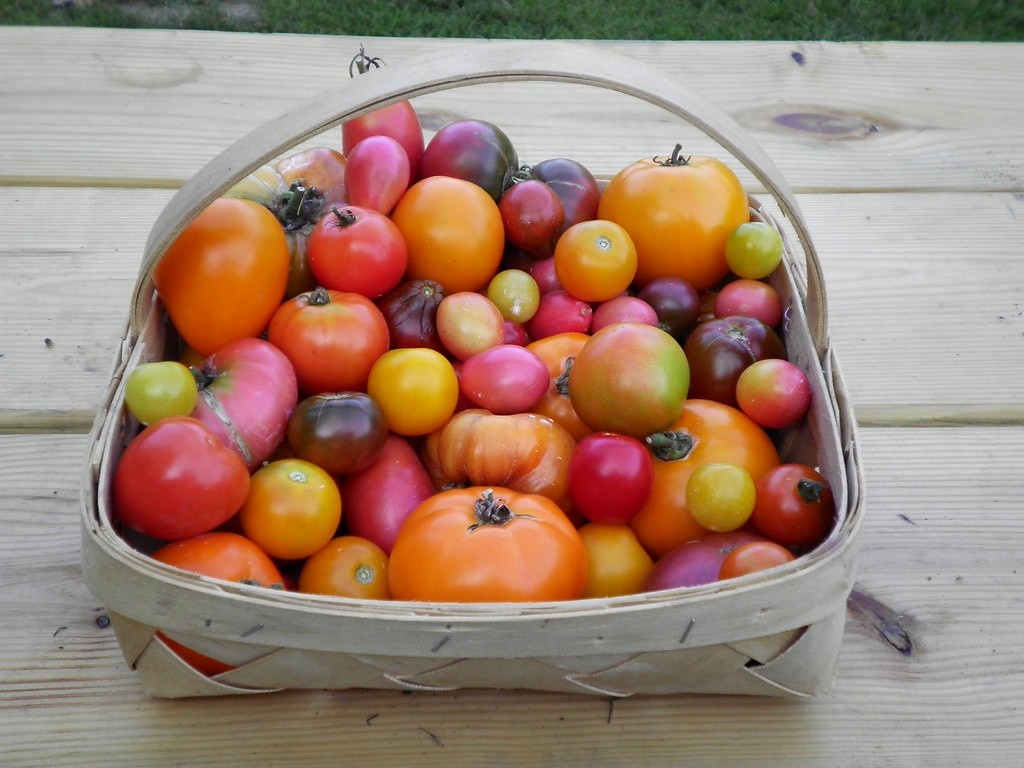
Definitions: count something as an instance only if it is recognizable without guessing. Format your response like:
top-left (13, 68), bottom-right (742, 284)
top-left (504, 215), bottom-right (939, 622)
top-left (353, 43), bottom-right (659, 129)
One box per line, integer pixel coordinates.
top-left (0, 25), bottom-right (1024, 768)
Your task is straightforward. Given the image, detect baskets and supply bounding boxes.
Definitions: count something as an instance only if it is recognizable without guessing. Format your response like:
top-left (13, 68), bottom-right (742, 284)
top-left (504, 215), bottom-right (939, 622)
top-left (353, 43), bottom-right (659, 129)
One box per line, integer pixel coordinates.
top-left (80, 41), bottom-right (866, 699)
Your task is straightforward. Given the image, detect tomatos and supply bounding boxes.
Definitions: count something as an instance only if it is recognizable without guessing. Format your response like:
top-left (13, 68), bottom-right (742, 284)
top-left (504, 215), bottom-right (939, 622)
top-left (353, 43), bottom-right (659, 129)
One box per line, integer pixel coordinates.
top-left (109, 44), bottom-right (835, 604)
